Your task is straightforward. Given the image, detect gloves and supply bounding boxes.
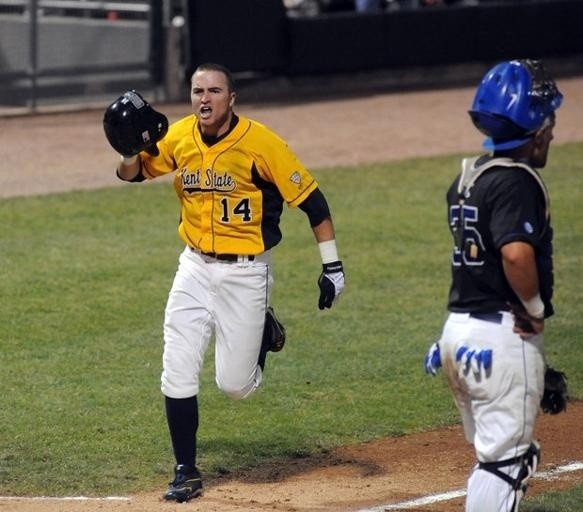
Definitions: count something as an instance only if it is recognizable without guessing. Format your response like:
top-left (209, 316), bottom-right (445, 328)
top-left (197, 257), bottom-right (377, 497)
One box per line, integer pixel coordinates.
top-left (454, 345), bottom-right (493, 376)
top-left (318, 260), bottom-right (346, 310)
top-left (424, 340), bottom-right (443, 377)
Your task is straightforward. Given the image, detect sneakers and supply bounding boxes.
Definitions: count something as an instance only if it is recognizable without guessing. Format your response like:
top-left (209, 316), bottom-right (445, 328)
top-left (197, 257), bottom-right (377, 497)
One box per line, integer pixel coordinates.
top-left (161, 464), bottom-right (204, 502)
top-left (266, 306), bottom-right (285, 352)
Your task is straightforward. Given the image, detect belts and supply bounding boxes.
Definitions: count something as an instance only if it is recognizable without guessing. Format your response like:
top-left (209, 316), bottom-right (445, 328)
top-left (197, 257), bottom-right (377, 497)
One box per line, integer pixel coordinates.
top-left (191, 246), bottom-right (255, 261)
top-left (471, 308), bottom-right (537, 336)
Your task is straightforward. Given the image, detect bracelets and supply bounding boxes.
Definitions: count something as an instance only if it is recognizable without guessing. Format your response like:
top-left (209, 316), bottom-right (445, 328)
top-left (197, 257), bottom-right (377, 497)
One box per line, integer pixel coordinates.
top-left (519, 292), bottom-right (546, 318)
top-left (119, 154), bottom-right (137, 167)
top-left (317, 238), bottom-right (338, 265)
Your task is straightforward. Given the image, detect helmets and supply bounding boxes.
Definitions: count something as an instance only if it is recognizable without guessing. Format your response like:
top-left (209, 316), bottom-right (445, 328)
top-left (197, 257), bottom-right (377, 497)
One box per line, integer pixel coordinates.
top-left (103, 89), bottom-right (169, 158)
top-left (466, 58), bottom-right (563, 152)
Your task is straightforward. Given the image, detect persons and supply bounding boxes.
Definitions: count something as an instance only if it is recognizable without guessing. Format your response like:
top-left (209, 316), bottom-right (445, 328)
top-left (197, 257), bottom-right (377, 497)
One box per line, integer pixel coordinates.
top-left (115, 62), bottom-right (346, 502)
top-left (422, 56), bottom-right (567, 511)
